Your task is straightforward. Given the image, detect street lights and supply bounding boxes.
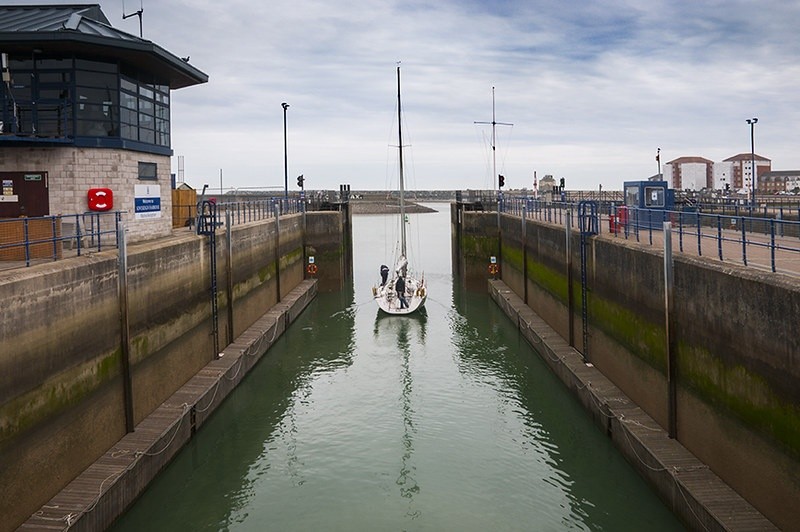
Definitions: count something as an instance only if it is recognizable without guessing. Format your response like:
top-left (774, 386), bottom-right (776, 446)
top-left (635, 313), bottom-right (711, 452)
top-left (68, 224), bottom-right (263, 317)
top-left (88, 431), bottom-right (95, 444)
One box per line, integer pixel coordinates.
top-left (747, 118), bottom-right (758, 210)
top-left (281, 102), bottom-right (290, 199)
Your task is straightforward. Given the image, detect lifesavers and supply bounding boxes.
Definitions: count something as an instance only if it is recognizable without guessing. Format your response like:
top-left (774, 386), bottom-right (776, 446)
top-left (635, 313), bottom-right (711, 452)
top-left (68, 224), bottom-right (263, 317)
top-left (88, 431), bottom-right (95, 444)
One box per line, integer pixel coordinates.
top-left (95, 191), bottom-right (107, 208)
top-left (307, 264), bottom-right (318, 275)
top-left (489, 264), bottom-right (499, 275)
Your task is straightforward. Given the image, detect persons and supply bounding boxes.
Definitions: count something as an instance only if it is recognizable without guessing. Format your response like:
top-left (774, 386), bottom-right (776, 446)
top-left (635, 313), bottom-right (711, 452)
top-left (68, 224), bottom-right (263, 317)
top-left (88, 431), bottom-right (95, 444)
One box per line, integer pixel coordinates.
top-left (395, 276), bottom-right (409, 309)
top-left (381, 266), bottom-right (389, 285)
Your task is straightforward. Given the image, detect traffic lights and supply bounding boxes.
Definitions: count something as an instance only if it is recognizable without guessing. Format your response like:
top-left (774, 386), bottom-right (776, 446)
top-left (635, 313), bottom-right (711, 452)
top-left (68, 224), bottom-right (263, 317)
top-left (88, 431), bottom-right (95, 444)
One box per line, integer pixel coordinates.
top-left (297, 175), bottom-right (304, 187)
top-left (726, 183), bottom-right (730, 191)
top-left (560, 178), bottom-right (564, 187)
top-left (499, 174), bottom-right (505, 187)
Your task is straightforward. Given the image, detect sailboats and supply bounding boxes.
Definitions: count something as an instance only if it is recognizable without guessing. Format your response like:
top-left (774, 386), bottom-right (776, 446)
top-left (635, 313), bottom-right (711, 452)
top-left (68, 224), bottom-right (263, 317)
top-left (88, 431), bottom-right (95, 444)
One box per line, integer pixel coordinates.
top-left (371, 61), bottom-right (429, 315)
